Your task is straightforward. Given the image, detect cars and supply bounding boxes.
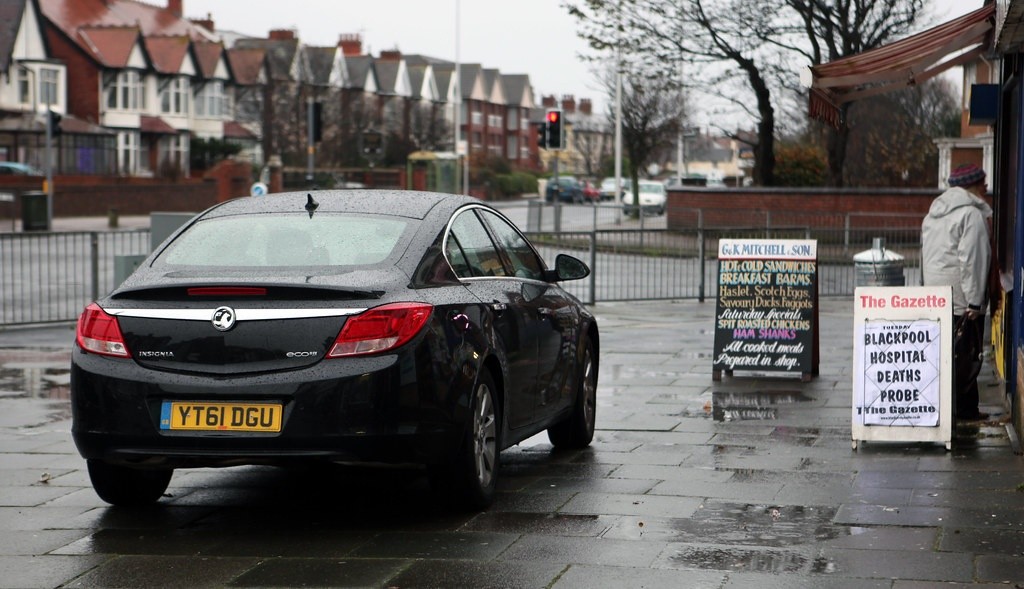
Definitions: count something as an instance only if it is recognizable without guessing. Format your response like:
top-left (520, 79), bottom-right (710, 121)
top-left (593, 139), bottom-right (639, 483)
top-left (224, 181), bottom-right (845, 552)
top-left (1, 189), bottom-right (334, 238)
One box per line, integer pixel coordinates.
top-left (621, 180), bottom-right (668, 216)
top-left (596, 177), bottom-right (628, 201)
top-left (579, 178), bottom-right (600, 202)
top-left (71, 189), bottom-right (600, 508)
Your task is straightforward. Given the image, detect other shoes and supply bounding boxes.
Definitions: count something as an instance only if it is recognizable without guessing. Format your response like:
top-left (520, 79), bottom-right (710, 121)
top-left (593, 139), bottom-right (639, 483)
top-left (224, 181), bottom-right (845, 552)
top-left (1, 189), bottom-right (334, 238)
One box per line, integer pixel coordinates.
top-left (956, 425), bottom-right (978, 436)
top-left (967, 413), bottom-right (989, 421)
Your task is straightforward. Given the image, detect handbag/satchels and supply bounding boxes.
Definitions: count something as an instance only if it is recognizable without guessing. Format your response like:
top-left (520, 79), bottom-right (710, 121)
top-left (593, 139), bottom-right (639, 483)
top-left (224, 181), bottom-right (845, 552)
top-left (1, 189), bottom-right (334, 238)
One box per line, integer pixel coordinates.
top-left (955, 310), bottom-right (984, 398)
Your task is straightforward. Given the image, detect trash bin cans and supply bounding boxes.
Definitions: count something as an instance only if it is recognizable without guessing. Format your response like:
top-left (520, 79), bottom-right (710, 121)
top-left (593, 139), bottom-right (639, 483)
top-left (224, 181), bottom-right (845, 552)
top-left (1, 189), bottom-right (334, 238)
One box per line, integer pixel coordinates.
top-left (21, 191), bottom-right (48, 231)
top-left (108, 209), bottom-right (118, 228)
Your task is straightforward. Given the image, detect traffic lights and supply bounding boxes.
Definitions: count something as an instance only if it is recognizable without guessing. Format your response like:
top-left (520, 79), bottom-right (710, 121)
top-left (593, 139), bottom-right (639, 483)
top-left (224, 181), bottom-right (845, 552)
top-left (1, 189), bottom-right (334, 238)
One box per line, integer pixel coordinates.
top-left (536, 122), bottom-right (546, 148)
top-left (544, 109), bottom-right (564, 150)
top-left (50, 112), bottom-right (63, 139)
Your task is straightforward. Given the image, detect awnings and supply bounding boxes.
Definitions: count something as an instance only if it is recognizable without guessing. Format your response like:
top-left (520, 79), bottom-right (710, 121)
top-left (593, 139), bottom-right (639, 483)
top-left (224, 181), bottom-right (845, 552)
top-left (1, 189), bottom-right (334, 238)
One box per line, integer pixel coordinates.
top-left (806, 0), bottom-right (996, 132)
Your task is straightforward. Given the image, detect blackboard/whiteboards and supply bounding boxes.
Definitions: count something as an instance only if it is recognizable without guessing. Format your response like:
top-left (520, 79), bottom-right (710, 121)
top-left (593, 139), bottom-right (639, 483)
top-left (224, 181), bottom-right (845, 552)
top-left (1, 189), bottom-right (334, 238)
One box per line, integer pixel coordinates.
top-left (713, 259), bottom-right (815, 373)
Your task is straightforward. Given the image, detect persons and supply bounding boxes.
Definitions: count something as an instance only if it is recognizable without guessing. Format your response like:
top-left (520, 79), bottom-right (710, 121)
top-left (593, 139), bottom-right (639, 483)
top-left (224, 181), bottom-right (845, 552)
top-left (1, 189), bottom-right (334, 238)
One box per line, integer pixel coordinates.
top-left (921, 163), bottom-right (992, 420)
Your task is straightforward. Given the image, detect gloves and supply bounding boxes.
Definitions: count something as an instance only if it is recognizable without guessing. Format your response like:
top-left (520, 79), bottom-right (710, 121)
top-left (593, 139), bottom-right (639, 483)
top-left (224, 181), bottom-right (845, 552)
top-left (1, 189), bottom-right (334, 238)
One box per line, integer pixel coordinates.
top-left (965, 303), bottom-right (981, 321)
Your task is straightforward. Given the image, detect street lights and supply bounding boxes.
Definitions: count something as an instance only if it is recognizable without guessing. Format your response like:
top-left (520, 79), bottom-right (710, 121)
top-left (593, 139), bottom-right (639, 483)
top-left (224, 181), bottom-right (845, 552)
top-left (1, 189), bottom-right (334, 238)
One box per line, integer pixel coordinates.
top-left (709, 122), bottom-right (739, 187)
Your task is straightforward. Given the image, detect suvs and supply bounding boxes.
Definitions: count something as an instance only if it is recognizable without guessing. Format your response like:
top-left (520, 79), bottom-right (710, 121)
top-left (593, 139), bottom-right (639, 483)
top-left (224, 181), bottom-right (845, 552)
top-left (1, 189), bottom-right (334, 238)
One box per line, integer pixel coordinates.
top-left (547, 176), bottom-right (585, 204)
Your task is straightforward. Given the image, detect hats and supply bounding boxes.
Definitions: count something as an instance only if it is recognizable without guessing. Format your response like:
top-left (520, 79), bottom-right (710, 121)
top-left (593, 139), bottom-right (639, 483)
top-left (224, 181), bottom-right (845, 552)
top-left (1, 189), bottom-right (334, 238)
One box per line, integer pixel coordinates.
top-left (948, 164), bottom-right (986, 187)
